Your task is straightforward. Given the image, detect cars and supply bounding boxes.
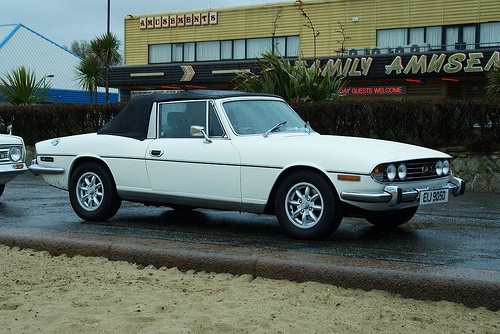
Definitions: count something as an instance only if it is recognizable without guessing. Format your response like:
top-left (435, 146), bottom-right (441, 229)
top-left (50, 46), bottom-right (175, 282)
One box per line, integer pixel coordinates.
top-left (28, 85), bottom-right (465, 240)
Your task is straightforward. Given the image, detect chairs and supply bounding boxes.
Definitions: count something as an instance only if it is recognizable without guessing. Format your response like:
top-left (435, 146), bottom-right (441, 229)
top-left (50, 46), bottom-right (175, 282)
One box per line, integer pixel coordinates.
top-left (164, 112), bottom-right (188, 137)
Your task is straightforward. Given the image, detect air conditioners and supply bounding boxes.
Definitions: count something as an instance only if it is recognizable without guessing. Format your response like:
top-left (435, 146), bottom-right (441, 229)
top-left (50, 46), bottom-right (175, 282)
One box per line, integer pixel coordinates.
top-left (348, 48), bottom-right (367, 56)
top-left (394, 45), bottom-right (410, 53)
top-left (409, 43), bottom-right (430, 52)
top-left (369, 46), bottom-right (389, 55)
